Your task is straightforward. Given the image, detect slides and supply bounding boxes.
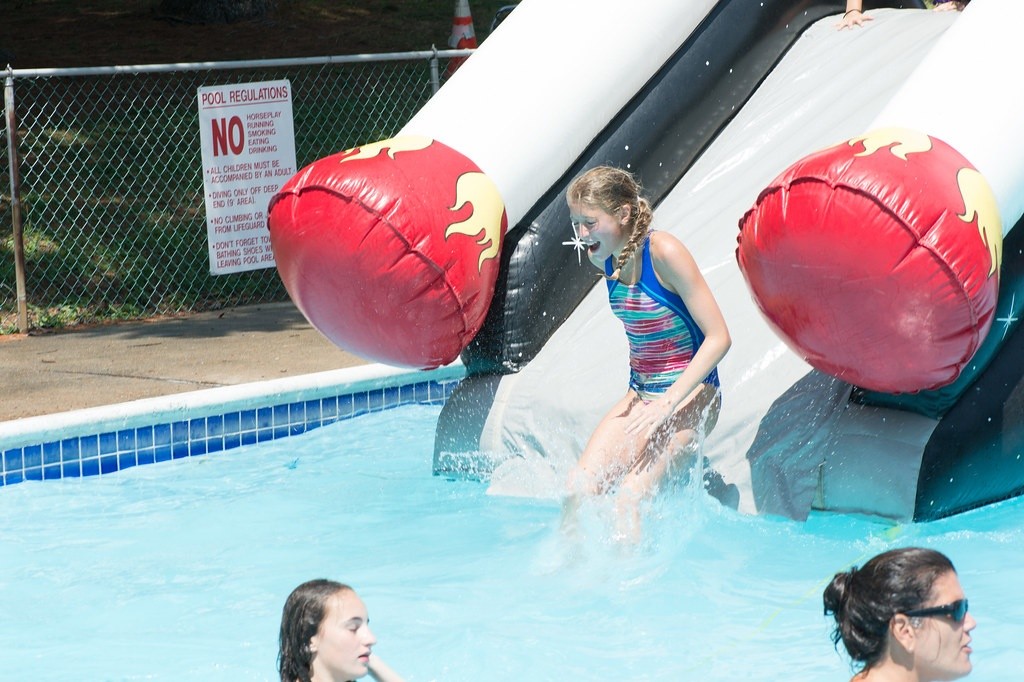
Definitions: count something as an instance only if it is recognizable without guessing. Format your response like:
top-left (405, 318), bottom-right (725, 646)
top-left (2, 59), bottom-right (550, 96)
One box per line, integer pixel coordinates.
top-left (266, 0), bottom-right (1024, 524)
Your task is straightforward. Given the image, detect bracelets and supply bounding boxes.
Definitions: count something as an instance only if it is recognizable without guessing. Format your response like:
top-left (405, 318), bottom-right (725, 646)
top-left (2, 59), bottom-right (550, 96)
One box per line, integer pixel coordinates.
top-left (842, 7), bottom-right (862, 18)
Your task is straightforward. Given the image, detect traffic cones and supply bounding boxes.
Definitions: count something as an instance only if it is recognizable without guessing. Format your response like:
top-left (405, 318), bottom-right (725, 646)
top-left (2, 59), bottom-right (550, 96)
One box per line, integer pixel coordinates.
top-left (444, 1), bottom-right (479, 82)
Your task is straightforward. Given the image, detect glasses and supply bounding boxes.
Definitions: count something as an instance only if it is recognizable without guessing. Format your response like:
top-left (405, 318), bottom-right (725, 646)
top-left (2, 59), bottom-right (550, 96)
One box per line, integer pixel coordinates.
top-left (898, 599), bottom-right (971, 619)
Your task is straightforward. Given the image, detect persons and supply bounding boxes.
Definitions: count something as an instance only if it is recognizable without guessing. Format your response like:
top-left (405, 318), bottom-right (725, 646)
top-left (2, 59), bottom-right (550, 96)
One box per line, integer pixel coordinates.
top-left (820, 545), bottom-right (976, 681)
top-left (544, 165), bottom-right (734, 525)
top-left (836, 0), bottom-right (971, 32)
top-left (275, 579), bottom-right (400, 682)
